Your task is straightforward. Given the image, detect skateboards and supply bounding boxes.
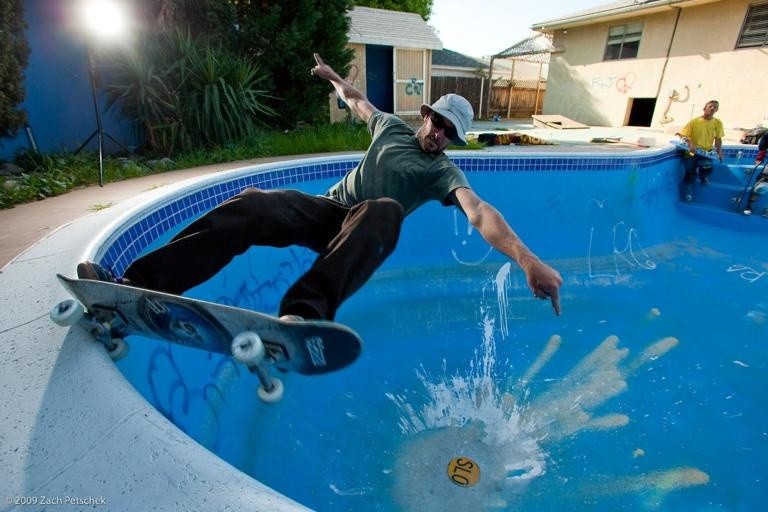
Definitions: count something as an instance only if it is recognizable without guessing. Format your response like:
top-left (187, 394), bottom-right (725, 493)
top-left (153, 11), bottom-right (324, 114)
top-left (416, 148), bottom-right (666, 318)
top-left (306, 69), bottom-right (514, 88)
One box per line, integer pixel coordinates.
top-left (51, 274), bottom-right (362, 403)
top-left (730, 161), bottom-right (762, 214)
top-left (670, 141), bottom-right (720, 160)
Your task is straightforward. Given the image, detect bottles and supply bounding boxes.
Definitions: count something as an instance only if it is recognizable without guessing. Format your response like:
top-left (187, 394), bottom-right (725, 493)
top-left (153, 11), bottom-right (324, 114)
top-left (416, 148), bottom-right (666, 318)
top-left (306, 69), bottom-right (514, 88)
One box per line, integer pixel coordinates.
top-left (737, 149), bottom-right (743, 164)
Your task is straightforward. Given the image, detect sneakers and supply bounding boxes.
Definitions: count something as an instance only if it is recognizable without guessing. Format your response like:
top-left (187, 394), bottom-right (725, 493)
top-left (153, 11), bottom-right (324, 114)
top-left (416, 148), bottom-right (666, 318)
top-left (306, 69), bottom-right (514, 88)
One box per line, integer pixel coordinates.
top-left (279, 312), bottom-right (311, 324)
top-left (685, 178), bottom-right (710, 202)
top-left (75, 259), bottom-right (132, 286)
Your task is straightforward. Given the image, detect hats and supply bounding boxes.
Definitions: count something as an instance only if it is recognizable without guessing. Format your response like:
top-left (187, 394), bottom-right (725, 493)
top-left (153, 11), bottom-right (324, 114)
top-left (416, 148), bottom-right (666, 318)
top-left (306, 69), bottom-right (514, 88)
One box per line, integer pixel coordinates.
top-left (419, 92), bottom-right (475, 147)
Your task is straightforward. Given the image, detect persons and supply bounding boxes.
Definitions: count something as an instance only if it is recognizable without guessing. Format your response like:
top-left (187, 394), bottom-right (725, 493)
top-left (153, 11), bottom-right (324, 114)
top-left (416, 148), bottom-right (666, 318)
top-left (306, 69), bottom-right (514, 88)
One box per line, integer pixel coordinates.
top-left (74, 52), bottom-right (566, 326)
top-left (673, 99), bottom-right (727, 204)
top-left (735, 129), bottom-right (768, 217)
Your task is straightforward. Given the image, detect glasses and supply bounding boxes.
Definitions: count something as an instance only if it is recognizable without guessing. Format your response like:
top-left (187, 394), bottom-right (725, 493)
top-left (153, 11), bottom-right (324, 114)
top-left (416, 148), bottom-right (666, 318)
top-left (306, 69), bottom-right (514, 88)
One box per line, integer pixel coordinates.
top-left (430, 110), bottom-right (459, 141)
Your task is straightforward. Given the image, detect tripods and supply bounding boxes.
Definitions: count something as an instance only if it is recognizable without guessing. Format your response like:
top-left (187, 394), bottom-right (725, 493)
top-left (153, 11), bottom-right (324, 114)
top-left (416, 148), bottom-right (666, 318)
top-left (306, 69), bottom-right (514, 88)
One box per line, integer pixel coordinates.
top-left (73, 43), bottom-right (155, 188)
